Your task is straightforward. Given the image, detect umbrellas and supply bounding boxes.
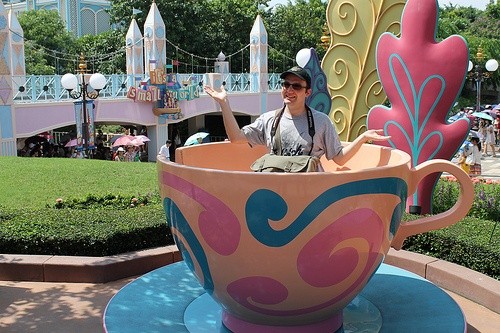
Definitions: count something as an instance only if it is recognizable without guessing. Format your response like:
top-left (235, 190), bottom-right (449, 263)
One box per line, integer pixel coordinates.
top-left (184, 132), bottom-right (209, 147)
top-left (112, 135), bottom-right (145, 148)
top-left (106, 135), bottom-right (123, 143)
top-left (472, 112), bottom-right (494, 122)
top-left (64, 138), bottom-right (85, 147)
top-left (449, 115), bottom-right (462, 122)
top-left (133, 135), bottom-right (150, 142)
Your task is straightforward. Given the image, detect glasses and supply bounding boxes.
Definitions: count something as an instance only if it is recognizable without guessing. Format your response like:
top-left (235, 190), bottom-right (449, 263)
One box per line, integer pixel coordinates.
top-left (280, 81), bottom-right (307, 90)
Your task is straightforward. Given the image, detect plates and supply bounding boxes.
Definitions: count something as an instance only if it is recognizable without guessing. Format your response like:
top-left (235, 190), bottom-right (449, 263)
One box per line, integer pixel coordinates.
top-left (103, 259), bottom-right (466, 333)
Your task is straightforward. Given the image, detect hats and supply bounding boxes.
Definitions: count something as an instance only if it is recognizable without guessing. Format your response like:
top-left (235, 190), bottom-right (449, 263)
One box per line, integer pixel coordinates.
top-left (116, 147), bottom-right (125, 153)
top-left (280, 66), bottom-right (311, 85)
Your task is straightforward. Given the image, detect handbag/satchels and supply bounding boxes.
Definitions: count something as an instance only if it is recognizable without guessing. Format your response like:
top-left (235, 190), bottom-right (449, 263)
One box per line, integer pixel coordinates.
top-left (250, 154), bottom-right (319, 172)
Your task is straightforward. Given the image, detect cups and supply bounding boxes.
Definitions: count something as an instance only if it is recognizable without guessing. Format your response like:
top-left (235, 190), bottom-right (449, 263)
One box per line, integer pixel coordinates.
top-left (157, 140), bottom-right (474, 333)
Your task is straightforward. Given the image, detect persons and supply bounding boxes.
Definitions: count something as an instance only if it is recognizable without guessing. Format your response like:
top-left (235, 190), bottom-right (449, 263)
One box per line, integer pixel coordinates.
top-left (17, 130), bottom-right (148, 162)
top-left (462, 134), bottom-right (481, 179)
top-left (203, 66), bottom-right (392, 173)
top-left (448, 103), bottom-right (500, 157)
top-left (159, 138), bottom-right (172, 161)
top-left (196, 137), bottom-right (203, 146)
